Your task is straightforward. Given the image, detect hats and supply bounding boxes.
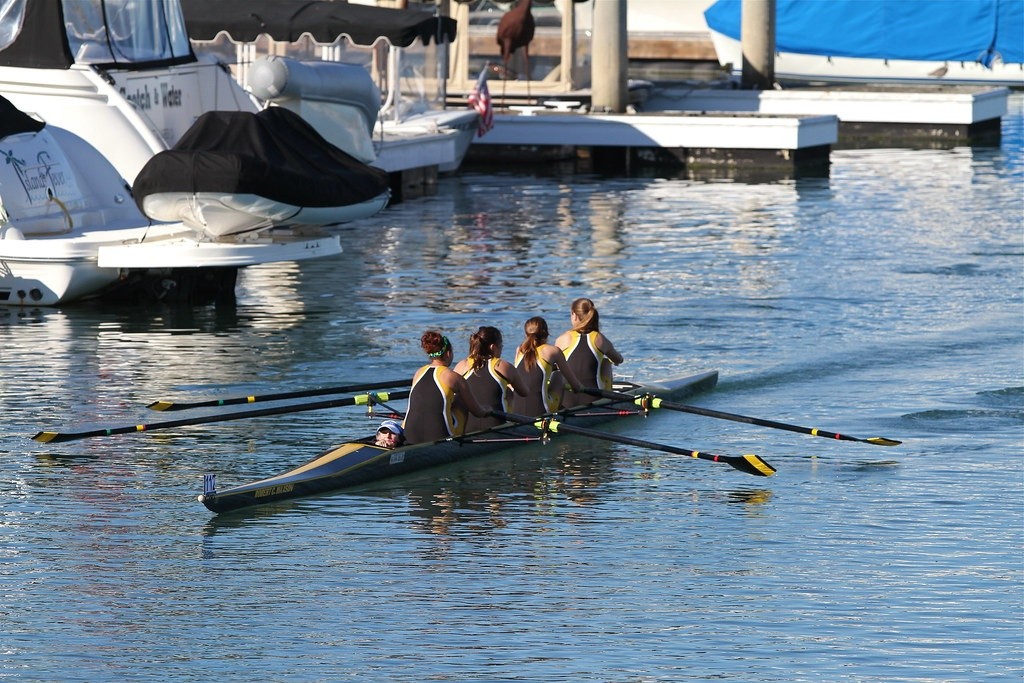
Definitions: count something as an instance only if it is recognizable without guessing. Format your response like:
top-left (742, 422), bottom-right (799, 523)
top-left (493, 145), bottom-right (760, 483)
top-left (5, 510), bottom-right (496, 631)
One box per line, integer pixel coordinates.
top-left (377, 420), bottom-right (402, 437)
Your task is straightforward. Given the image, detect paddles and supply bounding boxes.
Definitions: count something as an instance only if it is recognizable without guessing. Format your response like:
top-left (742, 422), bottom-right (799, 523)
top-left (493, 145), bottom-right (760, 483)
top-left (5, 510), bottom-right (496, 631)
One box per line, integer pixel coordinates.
top-left (143, 377), bottom-right (417, 414)
top-left (559, 383), bottom-right (904, 447)
top-left (484, 405), bottom-right (777, 478)
top-left (30, 383), bottom-right (412, 448)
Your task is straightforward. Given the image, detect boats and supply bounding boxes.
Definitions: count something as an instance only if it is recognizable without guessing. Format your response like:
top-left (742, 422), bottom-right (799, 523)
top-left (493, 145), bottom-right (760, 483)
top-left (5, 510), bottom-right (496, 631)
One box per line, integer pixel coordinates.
top-left (1, 0), bottom-right (494, 310)
top-left (705, 1), bottom-right (1024, 91)
top-left (195, 369), bottom-right (722, 518)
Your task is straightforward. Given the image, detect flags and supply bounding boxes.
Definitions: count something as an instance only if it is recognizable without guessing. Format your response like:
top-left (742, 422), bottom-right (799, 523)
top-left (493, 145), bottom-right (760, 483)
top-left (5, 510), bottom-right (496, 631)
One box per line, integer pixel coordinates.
top-left (466, 60), bottom-right (495, 137)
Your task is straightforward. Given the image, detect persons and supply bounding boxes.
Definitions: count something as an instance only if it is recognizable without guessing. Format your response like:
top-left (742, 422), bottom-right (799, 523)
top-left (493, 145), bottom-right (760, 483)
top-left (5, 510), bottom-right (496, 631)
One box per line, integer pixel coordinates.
top-left (555, 298), bottom-right (623, 410)
top-left (451, 326), bottom-right (529, 434)
top-left (374, 420), bottom-right (403, 449)
top-left (400, 331), bottom-right (494, 444)
top-left (506, 316), bottom-right (590, 418)
